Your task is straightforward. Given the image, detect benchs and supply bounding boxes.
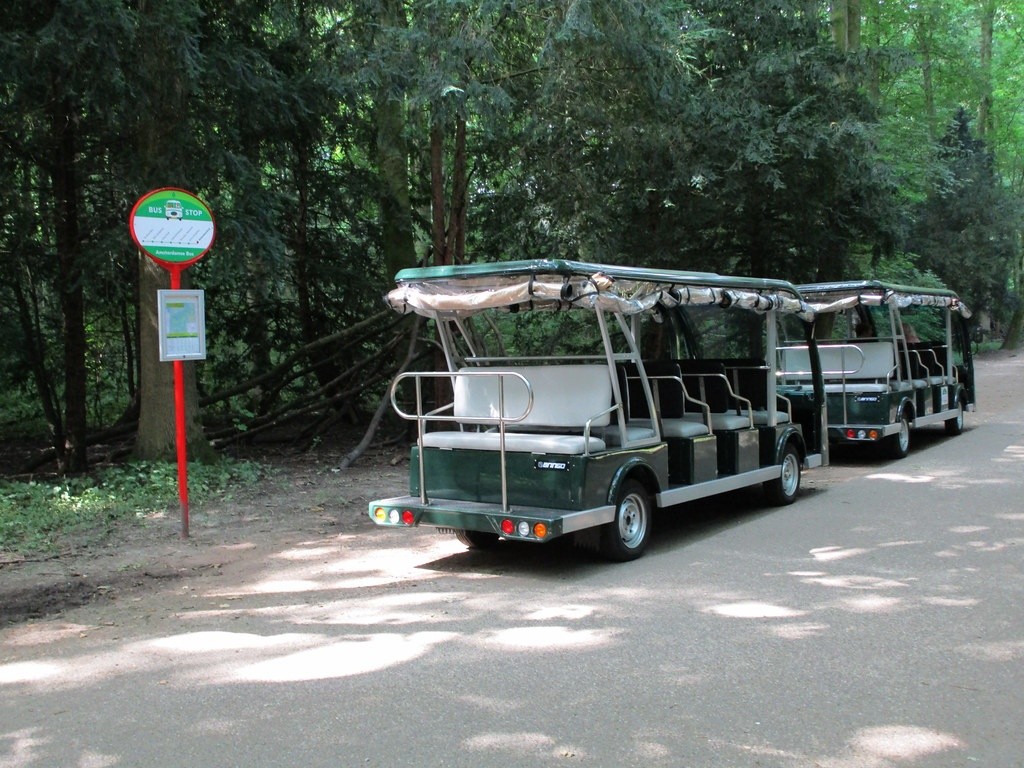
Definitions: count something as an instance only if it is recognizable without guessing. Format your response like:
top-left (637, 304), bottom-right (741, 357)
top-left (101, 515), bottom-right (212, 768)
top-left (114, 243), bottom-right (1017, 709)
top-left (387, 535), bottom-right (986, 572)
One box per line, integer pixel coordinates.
top-left (418, 339), bottom-right (958, 456)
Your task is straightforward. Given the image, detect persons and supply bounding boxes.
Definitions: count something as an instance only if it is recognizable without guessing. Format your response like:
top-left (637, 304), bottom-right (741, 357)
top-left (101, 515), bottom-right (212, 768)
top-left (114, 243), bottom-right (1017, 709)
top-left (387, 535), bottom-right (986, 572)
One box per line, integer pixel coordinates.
top-left (855, 322), bottom-right (873, 343)
top-left (897, 322), bottom-right (920, 344)
